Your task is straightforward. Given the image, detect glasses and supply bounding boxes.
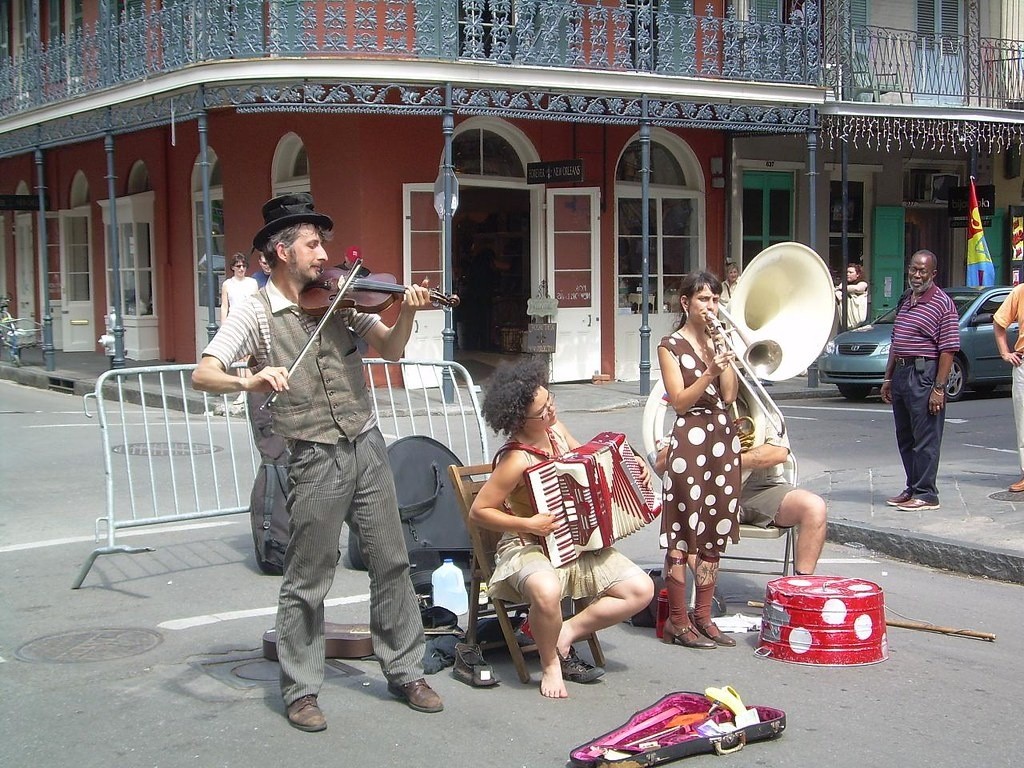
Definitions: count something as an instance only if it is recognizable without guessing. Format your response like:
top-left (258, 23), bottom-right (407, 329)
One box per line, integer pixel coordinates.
top-left (909, 266), bottom-right (934, 276)
top-left (233, 265), bottom-right (246, 268)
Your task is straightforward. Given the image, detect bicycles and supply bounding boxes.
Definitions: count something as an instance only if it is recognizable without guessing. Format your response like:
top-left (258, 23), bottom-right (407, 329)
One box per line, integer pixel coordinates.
top-left (0, 290), bottom-right (46, 368)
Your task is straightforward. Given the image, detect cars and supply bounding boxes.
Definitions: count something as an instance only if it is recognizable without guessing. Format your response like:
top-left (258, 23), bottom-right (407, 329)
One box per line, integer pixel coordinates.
top-left (817, 284), bottom-right (1020, 403)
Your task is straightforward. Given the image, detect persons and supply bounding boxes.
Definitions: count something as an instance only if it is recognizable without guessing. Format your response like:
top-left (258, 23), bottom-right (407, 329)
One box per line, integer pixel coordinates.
top-left (716, 262), bottom-right (739, 327)
top-left (657, 266), bottom-right (736, 650)
top-left (833, 262), bottom-right (868, 331)
top-left (191, 194), bottom-right (445, 732)
top-left (992, 282), bottom-right (1024, 492)
top-left (466, 358), bottom-right (652, 700)
top-left (220, 252), bottom-right (259, 403)
top-left (655, 404), bottom-right (827, 618)
top-left (332, 246), bottom-right (371, 393)
top-left (251, 252), bottom-right (270, 289)
top-left (881, 248), bottom-right (960, 511)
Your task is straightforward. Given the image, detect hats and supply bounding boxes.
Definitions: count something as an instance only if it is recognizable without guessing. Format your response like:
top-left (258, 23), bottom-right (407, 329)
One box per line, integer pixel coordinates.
top-left (251, 193), bottom-right (338, 252)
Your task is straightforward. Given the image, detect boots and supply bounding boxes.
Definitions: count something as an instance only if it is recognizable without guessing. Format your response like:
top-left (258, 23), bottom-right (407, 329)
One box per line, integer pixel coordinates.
top-left (664, 575), bottom-right (717, 648)
top-left (689, 579), bottom-right (737, 647)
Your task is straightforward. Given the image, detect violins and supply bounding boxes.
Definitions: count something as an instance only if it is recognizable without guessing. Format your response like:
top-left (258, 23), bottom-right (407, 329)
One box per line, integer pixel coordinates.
top-left (298, 261), bottom-right (462, 316)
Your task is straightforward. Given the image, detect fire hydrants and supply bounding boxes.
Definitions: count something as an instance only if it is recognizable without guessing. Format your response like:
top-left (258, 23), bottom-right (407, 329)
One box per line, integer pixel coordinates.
top-left (97, 309), bottom-right (128, 381)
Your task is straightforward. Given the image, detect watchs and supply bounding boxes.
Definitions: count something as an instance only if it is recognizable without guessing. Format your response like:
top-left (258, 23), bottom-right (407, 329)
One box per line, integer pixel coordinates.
top-left (931, 381), bottom-right (947, 389)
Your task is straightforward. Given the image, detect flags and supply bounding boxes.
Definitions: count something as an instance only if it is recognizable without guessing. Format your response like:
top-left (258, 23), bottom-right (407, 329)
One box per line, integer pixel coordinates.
top-left (966, 182), bottom-right (995, 289)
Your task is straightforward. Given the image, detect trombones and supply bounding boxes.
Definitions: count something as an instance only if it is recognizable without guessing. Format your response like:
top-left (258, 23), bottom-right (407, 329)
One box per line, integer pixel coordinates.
top-left (706, 312), bottom-right (785, 439)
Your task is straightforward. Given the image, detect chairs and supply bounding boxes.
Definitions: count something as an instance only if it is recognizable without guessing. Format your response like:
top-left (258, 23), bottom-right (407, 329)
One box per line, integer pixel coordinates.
top-left (449, 464), bottom-right (606, 684)
top-left (852, 52), bottom-right (904, 103)
top-left (718, 452), bottom-right (797, 607)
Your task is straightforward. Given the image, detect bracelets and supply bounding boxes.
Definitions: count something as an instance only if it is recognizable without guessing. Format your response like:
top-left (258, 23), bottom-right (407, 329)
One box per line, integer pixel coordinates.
top-left (933, 387), bottom-right (946, 397)
top-left (884, 379), bottom-right (892, 382)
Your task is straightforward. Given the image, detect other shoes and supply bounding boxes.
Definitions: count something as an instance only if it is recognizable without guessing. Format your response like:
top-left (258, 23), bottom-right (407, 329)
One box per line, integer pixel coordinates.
top-left (556, 643), bottom-right (606, 684)
top-left (234, 395), bottom-right (245, 406)
top-left (1008, 477), bottom-right (1024, 493)
top-left (886, 491), bottom-right (913, 506)
top-left (453, 642), bottom-right (500, 686)
top-left (387, 677), bottom-right (443, 712)
top-left (898, 496), bottom-right (939, 511)
top-left (287, 692), bottom-right (326, 731)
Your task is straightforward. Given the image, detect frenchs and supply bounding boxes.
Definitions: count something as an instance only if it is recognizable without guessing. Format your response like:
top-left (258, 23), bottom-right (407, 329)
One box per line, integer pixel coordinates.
top-left (641, 240), bottom-right (837, 487)
